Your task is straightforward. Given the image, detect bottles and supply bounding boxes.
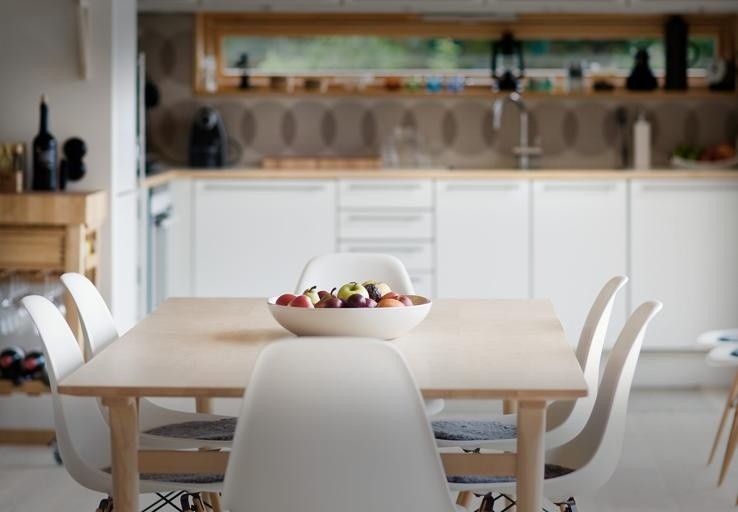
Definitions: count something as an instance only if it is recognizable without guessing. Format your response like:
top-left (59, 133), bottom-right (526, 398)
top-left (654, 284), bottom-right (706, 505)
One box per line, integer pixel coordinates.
top-left (32, 96), bottom-right (58, 192)
top-left (0, 346), bottom-right (50, 378)
top-left (9, 142), bottom-right (26, 195)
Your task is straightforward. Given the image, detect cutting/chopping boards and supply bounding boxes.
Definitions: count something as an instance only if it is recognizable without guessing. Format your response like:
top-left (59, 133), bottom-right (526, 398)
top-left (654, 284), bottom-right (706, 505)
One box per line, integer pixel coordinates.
top-left (258, 153), bottom-right (387, 170)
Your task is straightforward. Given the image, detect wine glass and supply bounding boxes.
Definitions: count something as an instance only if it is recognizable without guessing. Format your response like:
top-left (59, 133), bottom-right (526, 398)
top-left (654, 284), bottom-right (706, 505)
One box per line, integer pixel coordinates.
top-left (0, 267), bottom-right (69, 339)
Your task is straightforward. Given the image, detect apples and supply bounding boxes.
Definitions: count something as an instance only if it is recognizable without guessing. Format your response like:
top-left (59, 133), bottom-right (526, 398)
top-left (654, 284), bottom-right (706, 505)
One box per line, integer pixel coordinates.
top-left (276, 280), bottom-right (413, 308)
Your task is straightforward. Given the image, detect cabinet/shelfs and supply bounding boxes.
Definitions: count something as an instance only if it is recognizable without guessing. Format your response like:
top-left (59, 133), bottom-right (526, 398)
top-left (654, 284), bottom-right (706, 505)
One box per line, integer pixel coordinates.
top-left (3, 190), bottom-right (106, 446)
top-left (145, 161), bottom-right (738, 352)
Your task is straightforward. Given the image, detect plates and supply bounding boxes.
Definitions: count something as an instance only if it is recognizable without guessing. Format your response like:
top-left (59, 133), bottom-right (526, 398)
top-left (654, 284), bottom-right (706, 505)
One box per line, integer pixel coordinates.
top-left (668, 155), bottom-right (738, 172)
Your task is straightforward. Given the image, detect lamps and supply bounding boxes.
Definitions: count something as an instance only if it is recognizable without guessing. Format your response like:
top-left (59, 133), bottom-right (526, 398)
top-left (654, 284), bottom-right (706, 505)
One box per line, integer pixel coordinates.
top-left (488, 28), bottom-right (527, 93)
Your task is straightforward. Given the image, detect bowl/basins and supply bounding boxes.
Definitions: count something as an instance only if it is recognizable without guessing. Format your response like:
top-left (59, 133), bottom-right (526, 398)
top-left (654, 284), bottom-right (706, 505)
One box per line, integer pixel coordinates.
top-left (267, 288), bottom-right (432, 342)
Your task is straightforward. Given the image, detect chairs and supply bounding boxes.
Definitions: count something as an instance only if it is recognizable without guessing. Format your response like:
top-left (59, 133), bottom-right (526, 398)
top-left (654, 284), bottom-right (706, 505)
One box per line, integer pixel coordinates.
top-left (449, 301), bottom-right (662, 512)
top-left (433, 273), bottom-right (631, 507)
top-left (219, 338), bottom-right (453, 510)
top-left (58, 270), bottom-right (238, 510)
top-left (695, 328), bottom-right (737, 465)
top-left (708, 344), bottom-right (737, 487)
top-left (297, 250), bottom-right (447, 420)
top-left (19, 295), bottom-right (230, 511)
